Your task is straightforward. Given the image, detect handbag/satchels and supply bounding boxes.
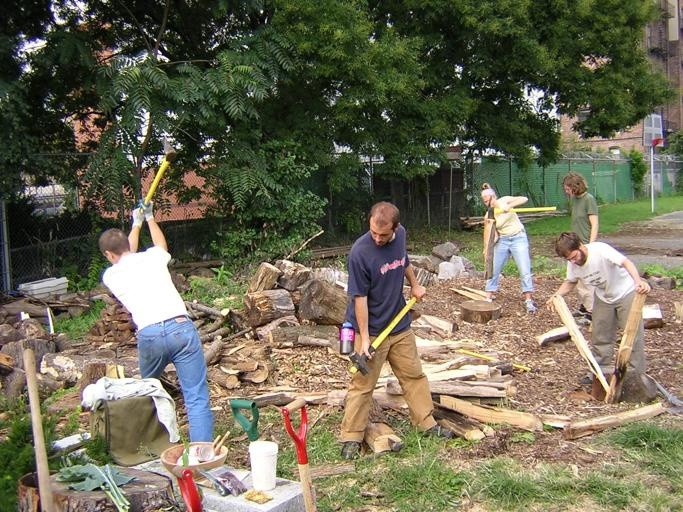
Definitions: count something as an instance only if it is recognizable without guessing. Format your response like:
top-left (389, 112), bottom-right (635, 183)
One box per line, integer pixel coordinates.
top-left (89, 396), bottom-right (179, 467)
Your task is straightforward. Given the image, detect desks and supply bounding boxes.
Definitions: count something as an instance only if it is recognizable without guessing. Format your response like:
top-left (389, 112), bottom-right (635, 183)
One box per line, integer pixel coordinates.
top-left (52, 432), bottom-right (316, 512)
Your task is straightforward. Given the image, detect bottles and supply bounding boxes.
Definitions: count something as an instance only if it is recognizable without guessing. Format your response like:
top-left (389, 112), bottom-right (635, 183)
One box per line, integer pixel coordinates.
top-left (339, 322), bottom-right (355, 355)
top-left (174, 445), bottom-right (215, 466)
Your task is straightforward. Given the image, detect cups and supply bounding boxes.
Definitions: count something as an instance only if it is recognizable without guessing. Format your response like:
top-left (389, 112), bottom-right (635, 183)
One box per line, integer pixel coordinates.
top-left (249, 440), bottom-right (279, 493)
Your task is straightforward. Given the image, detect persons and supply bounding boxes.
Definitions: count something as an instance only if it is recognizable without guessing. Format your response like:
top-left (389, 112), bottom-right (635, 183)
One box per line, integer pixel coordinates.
top-left (563, 172), bottom-right (599, 322)
top-left (98, 200), bottom-right (213, 441)
top-left (545, 232), bottom-right (651, 401)
top-left (338, 201), bottom-right (453, 464)
top-left (480, 182), bottom-right (537, 313)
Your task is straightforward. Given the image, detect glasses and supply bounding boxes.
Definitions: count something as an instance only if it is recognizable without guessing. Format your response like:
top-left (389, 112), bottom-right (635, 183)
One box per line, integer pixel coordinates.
top-left (566, 250), bottom-right (578, 261)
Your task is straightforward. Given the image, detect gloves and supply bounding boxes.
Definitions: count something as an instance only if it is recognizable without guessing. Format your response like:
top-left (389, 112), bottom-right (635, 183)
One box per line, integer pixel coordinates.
top-left (131, 202), bottom-right (153, 227)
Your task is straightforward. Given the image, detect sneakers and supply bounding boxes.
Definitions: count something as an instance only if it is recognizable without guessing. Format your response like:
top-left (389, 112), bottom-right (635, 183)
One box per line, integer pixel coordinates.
top-left (341, 440), bottom-right (360, 459)
top-left (525, 300), bottom-right (537, 314)
top-left (420, 424), bottom-right (455, 441)
top-left (572, 310), bottom-right (592, 326)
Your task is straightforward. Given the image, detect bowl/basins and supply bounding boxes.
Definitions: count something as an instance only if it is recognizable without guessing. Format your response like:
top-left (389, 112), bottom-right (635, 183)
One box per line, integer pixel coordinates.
top-left (159, 441), bottom-right (229, 480)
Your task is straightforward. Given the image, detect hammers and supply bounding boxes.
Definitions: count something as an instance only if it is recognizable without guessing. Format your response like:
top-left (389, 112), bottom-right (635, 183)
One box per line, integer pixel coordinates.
top-left (488, 205), bottom-right (556, 220)
top-left (349, 285), bottom-right (427, 377)
top-left (138, 136), bottom-right (177, 214)
